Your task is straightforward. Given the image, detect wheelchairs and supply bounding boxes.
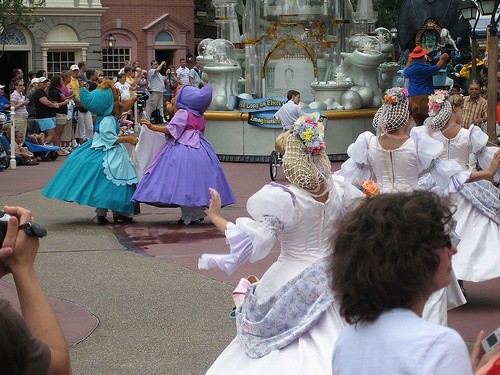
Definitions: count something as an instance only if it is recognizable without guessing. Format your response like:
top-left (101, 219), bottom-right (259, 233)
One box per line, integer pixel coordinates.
top-left (269, 147), bottom-right (286, 181)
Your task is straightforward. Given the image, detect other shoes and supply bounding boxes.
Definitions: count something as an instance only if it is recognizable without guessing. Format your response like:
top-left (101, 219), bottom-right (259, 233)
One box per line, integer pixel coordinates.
top-left (43, 127), bottom-right (133, 160)
top-left (26, 159), bottom-right (39, 165)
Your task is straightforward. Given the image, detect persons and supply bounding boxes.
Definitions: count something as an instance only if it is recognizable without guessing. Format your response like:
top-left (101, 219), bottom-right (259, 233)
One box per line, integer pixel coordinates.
top-left (199, 111), bottom-right (382, 375)
top-left (79, 80), bottom-right (140, 223)
top-left (0, 203), bottom-right (72, 375)
top-left (325, 187), bottom-right (472, 375)
top-left (332, 87), bottom-right (500, 200)
top-left (133, 84), bottom-right (236, 226)
top-left (417, 94), bottom-right (499, 308)
top-left (274, 89), bottom-right (307, 132)
top-left (402, 46), bottom-right (449, 125)
top-left (0, 55), bottom-right (207, 170)
top-left (458, 76), bottom-right (490, 130)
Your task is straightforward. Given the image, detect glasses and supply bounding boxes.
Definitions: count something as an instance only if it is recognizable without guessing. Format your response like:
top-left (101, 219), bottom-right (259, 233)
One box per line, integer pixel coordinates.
top-left (19, 85), bottom-right (25, 87)
top-left (432, 235), bottom-right (452, 250)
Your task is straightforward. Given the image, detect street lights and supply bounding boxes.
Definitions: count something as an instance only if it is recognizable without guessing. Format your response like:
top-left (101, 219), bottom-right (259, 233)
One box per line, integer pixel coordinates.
top-left (457, 0), bottom-right (482, 80)
top-left (475, 0), bottom-right (500, 150)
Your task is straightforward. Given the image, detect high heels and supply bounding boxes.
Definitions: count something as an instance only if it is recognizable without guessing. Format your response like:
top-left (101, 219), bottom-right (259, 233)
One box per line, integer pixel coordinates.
top-left (98, 216), bottom-right (108, 223)
top-left (113, 215), bottom-right (133, 222)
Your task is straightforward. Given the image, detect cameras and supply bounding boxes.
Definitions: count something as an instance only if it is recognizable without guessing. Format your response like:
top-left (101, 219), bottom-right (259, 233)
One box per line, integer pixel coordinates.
top-left (0, 212), bottom-right (13, 249)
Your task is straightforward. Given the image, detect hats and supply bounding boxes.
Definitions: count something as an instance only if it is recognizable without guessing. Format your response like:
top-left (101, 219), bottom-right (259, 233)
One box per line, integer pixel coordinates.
top-left (39, 77), bottom-right (49, 83)
top-left (0, 85), bottom-right (5, 89)
top-left (31, 78), bottom-right (39, 84)
top-left (409, 47), bottom-right (426, 58)
top-left (70, 65), bottom-right (79, 71)
